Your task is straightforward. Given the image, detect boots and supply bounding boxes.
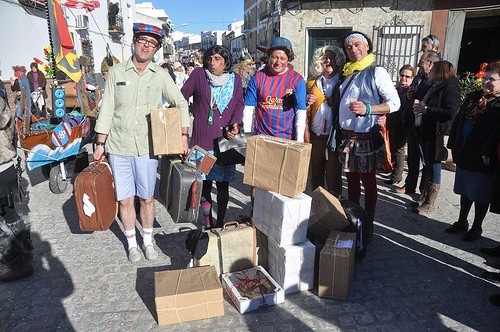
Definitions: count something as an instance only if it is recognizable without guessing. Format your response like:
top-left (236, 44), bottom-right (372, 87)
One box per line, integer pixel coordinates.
top-left (0, 231), bottom-right (33, 281)
top-left (411, 181), bottom-right (439, 213)
top-left (1, 219), bottom-right (32, 262)
top-left (410, 182), bottom-right (430, 207)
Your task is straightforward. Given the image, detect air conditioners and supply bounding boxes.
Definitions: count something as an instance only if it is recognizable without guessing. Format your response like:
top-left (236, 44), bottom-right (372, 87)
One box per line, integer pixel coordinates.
top-left (76, 14), bottom-right (89, 28)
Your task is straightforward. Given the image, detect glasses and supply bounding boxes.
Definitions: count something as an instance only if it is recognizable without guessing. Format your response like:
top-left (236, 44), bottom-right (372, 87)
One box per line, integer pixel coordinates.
top-left (136, 37), bottom-right (158, 48)
top-left (400, 74), bottom-right (411, 79)
top-left (481, 76), bottom-right (499, 83)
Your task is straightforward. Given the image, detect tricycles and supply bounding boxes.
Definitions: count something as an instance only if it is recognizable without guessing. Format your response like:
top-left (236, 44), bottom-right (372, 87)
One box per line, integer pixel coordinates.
top-left (15, 78), bottom-right (100, 193)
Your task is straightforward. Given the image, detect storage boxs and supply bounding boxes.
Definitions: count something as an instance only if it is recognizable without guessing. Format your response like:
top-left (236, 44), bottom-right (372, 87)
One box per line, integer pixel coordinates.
top-left (221, 265), bottom-right (285, 315)
top-left (242, 133), bottom-right (312, 198)
top-left (149, 106), bottom-right (184, 156)
top-left (252, 187), bottom-right (312, 247)
top-left (305, 185), bottom-right (351, 241)
top-left (184, 145), bottom-right (218, 175)
top-left (318, 231), bottom-right (358, 301)
top-left (267, 236), bottom-right (316, 295)
top-left (154, 265), bottom-right (225, 326)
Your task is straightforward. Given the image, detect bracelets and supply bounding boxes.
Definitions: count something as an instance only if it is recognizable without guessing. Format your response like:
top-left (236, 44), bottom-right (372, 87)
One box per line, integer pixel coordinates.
top-left (363, 100), bottom-right (371, 117)
top-left (182, 133), bottom-right (189, 137)
top-left (96, 141), bottom-right (105, 147)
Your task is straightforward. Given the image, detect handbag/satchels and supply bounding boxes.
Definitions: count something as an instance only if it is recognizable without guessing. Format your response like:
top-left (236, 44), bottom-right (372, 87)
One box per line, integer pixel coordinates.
top-left (218, 122), bottom-right (247, 164)
top-left (330, 191), bottom-right (368, 260)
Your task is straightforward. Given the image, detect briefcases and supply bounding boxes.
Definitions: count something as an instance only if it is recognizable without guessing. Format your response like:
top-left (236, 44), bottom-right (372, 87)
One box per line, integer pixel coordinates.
top-left (159, 153), bottom-right (203, 223)
top-left (73, 156), bottom-right (117, 232)
top-left (193, 221), bottom-right (258, 280)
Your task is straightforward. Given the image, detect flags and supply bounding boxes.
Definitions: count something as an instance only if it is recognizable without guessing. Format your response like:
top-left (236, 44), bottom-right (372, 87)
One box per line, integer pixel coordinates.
top-left (48, 0), bottom-right (96, 91)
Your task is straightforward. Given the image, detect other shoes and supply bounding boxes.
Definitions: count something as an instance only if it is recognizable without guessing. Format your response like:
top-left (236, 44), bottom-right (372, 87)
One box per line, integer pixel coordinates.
top-left (445, 221), bottom-right (469, 232)
top-left (480, 244), bottom-right (500, 258)
top-left (394, 185), bottom-right (414, 194)
top-left (142, 243), bottom-right (158, 260)
top-left (460, 227), bottom-right (482, 242)
top-left (128, 245), bottom-right (142, 262)
top-left (385, 179), bottom-right (401, 184)
top-left (484, 270), bottom-right (500, 283)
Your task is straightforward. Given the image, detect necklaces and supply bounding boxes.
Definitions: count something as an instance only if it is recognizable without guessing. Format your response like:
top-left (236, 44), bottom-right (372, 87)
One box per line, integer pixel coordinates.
top-left (209, 87), bottom-right (221, 110)
top-left (321, 78), bottom-right (328, 99)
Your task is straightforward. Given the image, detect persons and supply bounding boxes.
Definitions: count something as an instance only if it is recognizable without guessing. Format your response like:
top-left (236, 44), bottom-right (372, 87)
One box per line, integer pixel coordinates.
top-left (91, 57), bottom-right (120, 115)
top-left (305, 44), bottom-right (346, 197)
top-left (335, 31), bottom-right (402, 243)
top-left (161, 49), bottom-right (294, 138)
top-left (481, 243), bottom-right (500, 307)
top-left (180, 45), bottom-right (243, 231)
top-left (92, 22), bottom-right (188, 262)
top-left (444, 61), bottom-right (500, 241)
top-left (0, 63), bottom-right (48, 121)
top-left (242, 37), bottom-right (308, 225)
top-left (385, 34), bottom-right (461, 213)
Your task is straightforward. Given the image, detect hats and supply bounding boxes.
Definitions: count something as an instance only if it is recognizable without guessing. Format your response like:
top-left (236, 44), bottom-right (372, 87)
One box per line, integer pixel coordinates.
top-left (132, 23), bottom-right (164, 40)
top-left (269, 37), bottom-right (291, 54)
top-left (342, 31), bottom-right (373, 54)
